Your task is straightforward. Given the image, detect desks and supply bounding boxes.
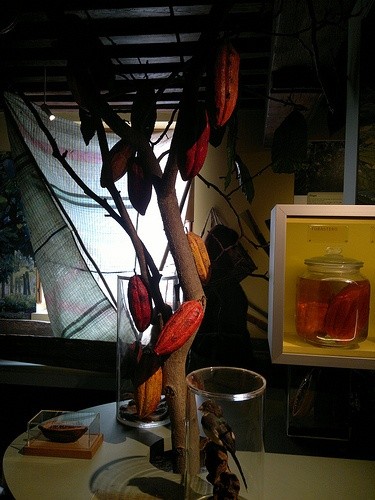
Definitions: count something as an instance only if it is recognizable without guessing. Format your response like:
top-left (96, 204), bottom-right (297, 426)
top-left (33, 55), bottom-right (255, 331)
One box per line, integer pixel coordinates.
top-left (2, 392), bottom-right (375, 500)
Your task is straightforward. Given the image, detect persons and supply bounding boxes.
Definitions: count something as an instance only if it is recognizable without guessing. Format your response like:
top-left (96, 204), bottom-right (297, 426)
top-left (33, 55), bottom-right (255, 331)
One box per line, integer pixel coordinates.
top-left (194, 225), bottom-right (250, 365)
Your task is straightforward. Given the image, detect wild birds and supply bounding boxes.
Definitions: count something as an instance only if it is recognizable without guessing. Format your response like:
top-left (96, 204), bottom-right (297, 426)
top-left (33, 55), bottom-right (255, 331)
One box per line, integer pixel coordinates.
top-left (198, 399), bottom-right (248, 491)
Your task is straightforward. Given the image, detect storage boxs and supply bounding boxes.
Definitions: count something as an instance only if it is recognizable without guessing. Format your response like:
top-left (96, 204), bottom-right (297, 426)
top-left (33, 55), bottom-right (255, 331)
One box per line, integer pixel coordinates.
top-left (23, 410), bottom-right (104, 459)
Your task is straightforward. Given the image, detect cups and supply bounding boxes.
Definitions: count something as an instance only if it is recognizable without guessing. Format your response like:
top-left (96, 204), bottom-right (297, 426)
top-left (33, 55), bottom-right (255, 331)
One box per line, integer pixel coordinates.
top-left (184, 365), bottom-right (269, 500)
top-left (116, 274), bottom-right (178, 429)
